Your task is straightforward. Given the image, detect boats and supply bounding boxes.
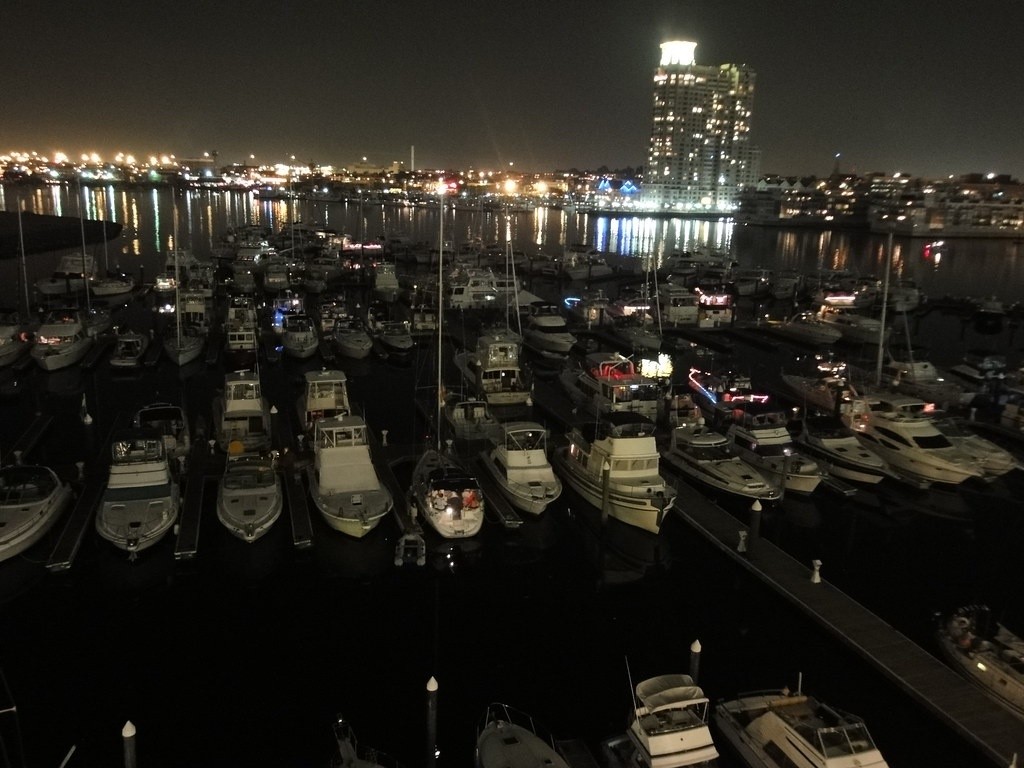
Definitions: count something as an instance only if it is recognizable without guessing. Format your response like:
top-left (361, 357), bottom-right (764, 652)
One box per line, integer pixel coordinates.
top-left (597, 634), bottom-right (723, 767)
top-left (474, 701), bottom-right (574, 768)
top-left (711, 671), bottom-right (890, 768)
top-left (932, 603), bottom-right (1024, 720)
top-left (0, 191), bottom-right (1024, 559)
